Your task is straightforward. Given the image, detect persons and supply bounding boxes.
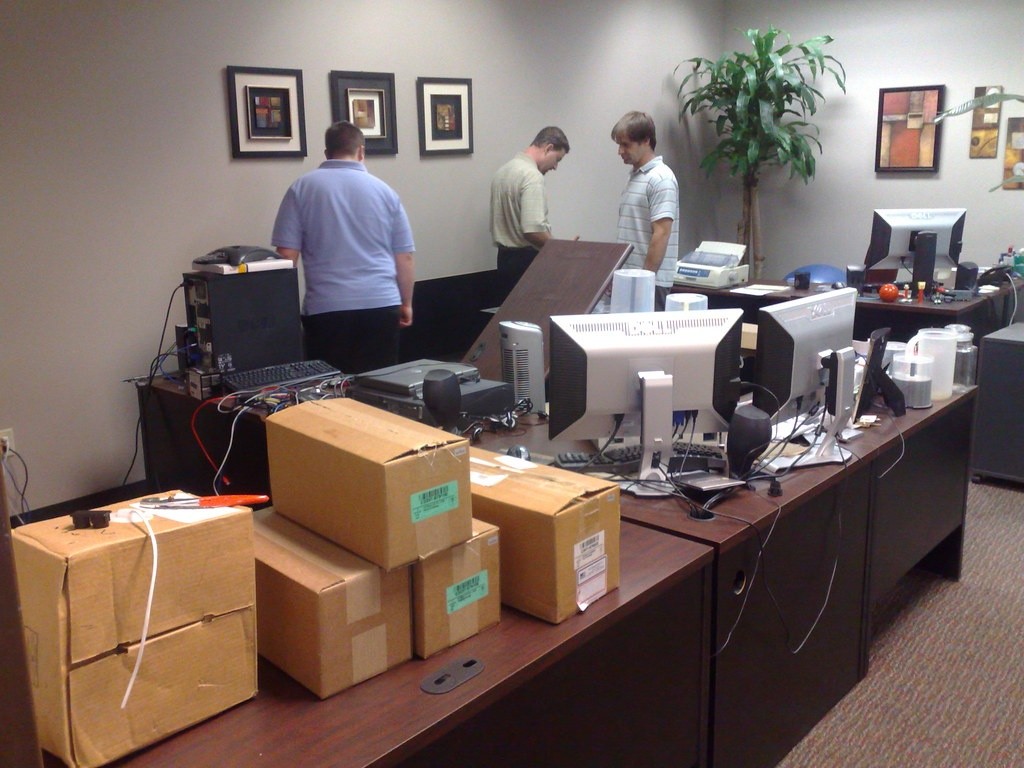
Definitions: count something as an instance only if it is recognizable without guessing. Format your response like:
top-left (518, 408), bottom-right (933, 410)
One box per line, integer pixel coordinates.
top-left (610, 110), bottom-right (679, 312)
top-left (490, 126), bottom-right (570, 308)
top-left (270, 121), bottom-right (414, 373)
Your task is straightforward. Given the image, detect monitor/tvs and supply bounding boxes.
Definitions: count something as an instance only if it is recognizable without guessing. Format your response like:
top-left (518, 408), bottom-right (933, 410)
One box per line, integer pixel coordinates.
top-left (547, 308), bottom-right (744, 497)
top-left (863, 207), bottom-right (967, 298)
top-left (751, 286), bottom-right (855, 471)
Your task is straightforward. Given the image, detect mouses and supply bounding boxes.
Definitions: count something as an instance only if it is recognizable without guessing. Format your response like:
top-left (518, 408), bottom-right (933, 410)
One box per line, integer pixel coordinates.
top-left (506, 443), bottom-right (531, 461)
top-left (831, 281), bottom-right (846, 288)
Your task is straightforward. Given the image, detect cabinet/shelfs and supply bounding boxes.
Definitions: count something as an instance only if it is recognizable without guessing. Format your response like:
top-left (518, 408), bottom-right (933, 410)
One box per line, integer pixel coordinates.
top-left (43, 371), bottom-right (976, 768)
top-left (968, 322), bottom-right (1024, 488)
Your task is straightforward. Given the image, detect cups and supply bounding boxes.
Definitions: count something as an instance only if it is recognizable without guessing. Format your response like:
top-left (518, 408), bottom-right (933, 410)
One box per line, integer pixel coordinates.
top-left (794, 272), bottom-right (811, 289)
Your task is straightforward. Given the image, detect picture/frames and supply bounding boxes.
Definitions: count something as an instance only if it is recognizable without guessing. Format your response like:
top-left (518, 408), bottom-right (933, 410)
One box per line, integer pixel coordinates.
top-left (417, 77), bottom-right (474, 157)
top-left (874, 84), bottom-right (944, 174)
top-left (227, 66), bottom-right (307, 159)
top-left (330, 70), bottom-right (398, 156)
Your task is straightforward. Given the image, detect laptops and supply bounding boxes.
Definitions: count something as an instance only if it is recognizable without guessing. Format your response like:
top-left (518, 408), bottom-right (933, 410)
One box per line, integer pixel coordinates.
top-left (355, 360), bottom-right (479, 396)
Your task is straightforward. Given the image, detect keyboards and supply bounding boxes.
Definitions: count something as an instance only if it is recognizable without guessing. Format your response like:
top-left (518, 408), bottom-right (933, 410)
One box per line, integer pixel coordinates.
top-left (221, 360), bottom-right (341, 394)
top-left (554, 440), bottom-right (728, 473)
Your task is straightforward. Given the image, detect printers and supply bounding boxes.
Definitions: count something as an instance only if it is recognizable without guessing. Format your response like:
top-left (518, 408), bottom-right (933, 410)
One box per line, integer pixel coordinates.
top-left (673, 250), bottom-right (749, 290)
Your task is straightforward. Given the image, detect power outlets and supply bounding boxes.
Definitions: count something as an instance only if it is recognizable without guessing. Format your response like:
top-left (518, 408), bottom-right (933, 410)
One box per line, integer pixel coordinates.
top-left (0, 429), bottom-right (16, 457)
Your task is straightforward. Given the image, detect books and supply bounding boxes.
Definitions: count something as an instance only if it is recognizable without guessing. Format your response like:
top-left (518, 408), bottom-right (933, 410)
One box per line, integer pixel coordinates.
top-left (191, 257), bottom-right (293, 275)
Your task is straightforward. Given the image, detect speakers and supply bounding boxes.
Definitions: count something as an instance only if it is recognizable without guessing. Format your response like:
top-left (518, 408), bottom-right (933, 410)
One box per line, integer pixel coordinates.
top-left (846, 264), bottom-right (864, 296)
top-left (727, 403), bottom-right (772, 490)
top-left (423, 369), bottom-right (462, 434)
top-left (952, 261), bottom-right (979, 300)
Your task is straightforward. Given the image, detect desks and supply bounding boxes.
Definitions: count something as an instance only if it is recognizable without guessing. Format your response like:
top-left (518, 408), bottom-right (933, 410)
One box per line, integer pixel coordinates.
top-left (671, 281), bottom-right (1024, 353)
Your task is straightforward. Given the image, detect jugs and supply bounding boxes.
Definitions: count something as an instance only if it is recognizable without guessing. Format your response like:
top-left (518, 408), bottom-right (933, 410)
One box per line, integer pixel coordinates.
top-left (904, 328), bottom-right (958, 402)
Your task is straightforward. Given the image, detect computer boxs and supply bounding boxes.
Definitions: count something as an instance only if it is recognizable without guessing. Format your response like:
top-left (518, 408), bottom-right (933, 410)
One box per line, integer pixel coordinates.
top-left (180, 270), bottom-right (304, 375)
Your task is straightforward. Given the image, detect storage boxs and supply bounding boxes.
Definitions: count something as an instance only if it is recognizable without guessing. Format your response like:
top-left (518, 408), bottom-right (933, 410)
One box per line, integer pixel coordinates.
top-left (412, 517), bottom-right (501, 660)
top-left (11, 490), bottom-right (258, 768)
top-left (468, 446), bottom-right (621, 625)
top-left (266, 398), bottom-right (473, 573)
top-left (253, 507), bottom-right (412, 700)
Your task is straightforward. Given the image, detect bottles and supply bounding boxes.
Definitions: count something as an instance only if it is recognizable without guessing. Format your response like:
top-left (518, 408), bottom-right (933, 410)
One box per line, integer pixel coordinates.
top-left (945, 323), bottom-right (978, 393)
